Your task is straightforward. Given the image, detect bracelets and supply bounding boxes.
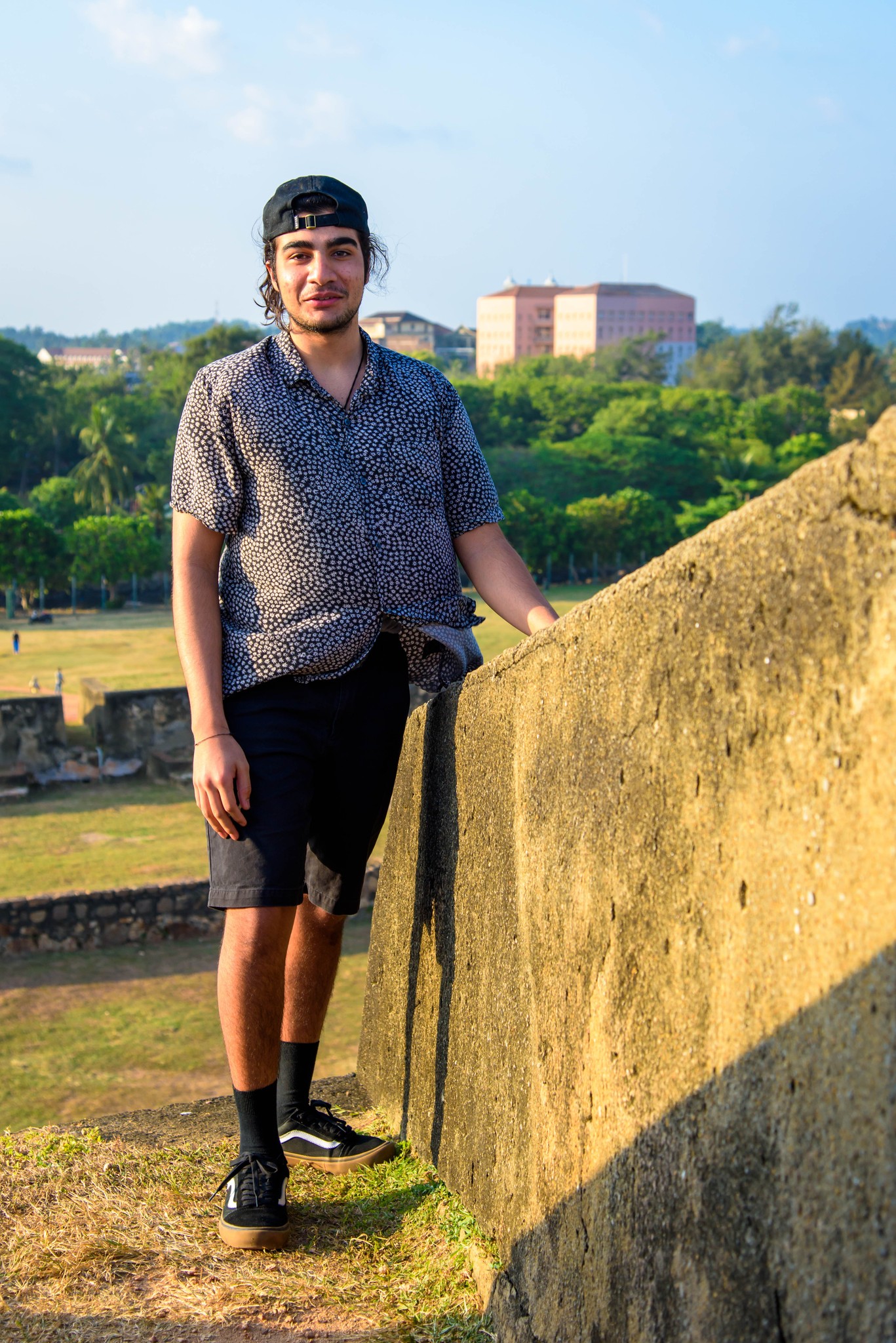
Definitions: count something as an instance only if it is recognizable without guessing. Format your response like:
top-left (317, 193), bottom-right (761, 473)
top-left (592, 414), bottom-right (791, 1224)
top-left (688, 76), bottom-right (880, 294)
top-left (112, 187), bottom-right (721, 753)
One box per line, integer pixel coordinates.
top-left (194, 733), bottom-right (232, 748)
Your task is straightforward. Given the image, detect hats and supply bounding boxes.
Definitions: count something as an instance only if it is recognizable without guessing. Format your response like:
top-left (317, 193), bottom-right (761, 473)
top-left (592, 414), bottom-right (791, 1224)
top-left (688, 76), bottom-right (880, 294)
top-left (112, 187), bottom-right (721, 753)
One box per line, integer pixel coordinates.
top-left (262, 175), bottom-right (370, 242)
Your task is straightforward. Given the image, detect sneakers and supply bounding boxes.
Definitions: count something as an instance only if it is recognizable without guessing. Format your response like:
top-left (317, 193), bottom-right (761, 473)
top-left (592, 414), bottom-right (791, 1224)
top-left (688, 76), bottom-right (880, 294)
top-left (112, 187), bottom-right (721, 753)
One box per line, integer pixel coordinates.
top-left (277, 1099), bottom-right (395, 1175)
top-left (209, 1151), bottom-right (290, 1249)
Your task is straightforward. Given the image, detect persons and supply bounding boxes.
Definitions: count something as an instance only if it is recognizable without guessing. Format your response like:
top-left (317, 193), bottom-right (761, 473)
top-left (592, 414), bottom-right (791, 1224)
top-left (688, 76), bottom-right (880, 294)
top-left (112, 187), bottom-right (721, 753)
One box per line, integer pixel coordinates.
top-left (12, 630), bottom-right (20, 652)
top-left (29, 674), bottom-right (40, 697)
top-left (54, 668), bottom-right (63, 697)
top-left (173, 174), bottom-right (561, 1251)
